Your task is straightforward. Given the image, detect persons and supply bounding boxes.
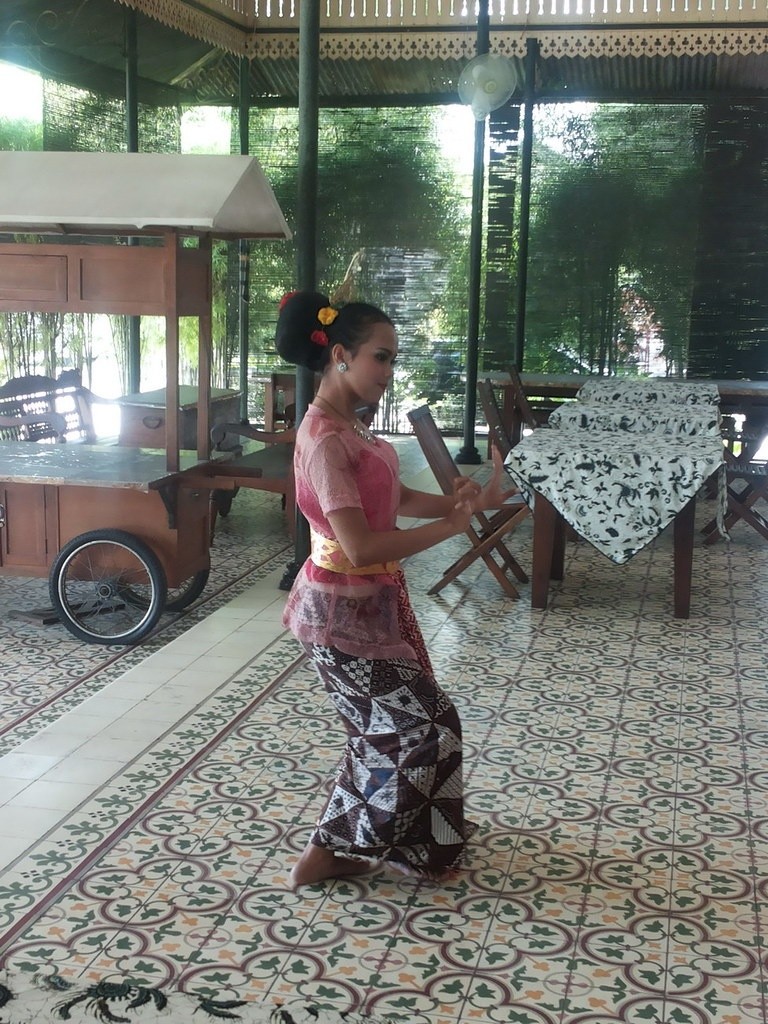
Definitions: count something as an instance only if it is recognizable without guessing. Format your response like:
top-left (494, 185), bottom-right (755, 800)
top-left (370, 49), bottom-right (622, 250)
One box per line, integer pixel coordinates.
top-left (275, 292), bottom-right (519, 884)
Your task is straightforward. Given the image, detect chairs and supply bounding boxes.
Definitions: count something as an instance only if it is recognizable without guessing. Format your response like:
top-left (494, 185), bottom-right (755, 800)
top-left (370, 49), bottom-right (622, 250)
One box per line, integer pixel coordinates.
top-left (264, 370), bottom-right (325, 447)
top-left (405, 364), bottom-right (768, 599)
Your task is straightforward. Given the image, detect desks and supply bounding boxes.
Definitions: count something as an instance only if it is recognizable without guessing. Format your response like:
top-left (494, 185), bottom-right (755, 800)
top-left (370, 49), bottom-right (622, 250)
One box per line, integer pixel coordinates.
top-left (549, 400), bottom-right (724, 435)
top-left (458, 369), bottom-right (768, 446)
top-left (504, 427), bottom-right (732, 619)
top-left (116, 384), bottom-right (244, 452)
top-left (574, 377), bottom-right (721, 405)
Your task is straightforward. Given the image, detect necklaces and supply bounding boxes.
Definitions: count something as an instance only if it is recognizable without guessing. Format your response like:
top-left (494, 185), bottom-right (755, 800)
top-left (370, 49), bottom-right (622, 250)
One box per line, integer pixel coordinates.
top-left (315, 395), bottom-right (376, 445)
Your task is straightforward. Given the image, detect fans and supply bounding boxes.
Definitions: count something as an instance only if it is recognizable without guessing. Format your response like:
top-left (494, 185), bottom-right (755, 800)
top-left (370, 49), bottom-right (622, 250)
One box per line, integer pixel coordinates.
top-left (458, 53), bottom-right (518, 119)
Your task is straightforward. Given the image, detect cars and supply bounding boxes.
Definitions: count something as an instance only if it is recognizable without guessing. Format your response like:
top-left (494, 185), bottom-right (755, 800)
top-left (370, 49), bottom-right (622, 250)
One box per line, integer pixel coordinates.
top-left (414, 350), bottom-right (466, 402)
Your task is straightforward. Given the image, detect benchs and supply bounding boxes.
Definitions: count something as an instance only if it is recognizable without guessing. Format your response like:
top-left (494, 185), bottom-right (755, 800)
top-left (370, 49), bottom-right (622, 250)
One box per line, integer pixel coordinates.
top-left (0, 369), bottom-right (120, 448)
top-left (210, 401), bottom-right (378, 549)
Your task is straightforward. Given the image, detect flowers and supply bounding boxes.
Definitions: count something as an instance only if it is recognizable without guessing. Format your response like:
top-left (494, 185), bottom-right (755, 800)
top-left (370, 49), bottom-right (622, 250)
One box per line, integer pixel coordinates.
top-left (279, 290), bottom-right (341, 348)
